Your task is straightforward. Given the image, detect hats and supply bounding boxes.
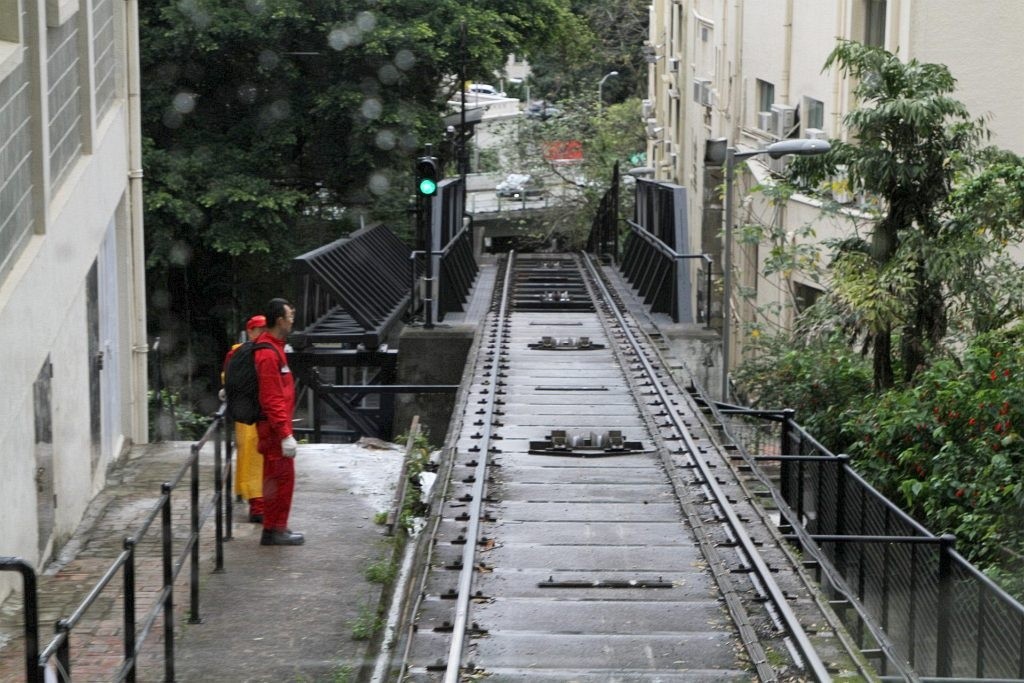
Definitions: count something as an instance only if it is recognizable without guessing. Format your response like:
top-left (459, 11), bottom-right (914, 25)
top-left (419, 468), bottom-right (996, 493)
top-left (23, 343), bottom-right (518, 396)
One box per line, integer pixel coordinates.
top-left (247, 316), bottom-right (266, 329)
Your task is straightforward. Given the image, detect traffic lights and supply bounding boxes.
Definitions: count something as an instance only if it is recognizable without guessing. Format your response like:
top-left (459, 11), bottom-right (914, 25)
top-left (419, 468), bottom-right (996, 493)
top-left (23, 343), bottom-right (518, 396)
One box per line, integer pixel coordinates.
top-left (417, 158), bottom-right (441, 198)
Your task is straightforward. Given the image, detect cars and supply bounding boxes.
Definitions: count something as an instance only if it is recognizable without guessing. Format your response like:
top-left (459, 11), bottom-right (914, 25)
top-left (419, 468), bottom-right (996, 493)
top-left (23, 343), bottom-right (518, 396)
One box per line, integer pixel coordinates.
top-left (467, 83), bottom-right (509, 99)
top-left (496, 172), bottom-right (548, 202)
top-left (521, 102), bottom-right (565, 122)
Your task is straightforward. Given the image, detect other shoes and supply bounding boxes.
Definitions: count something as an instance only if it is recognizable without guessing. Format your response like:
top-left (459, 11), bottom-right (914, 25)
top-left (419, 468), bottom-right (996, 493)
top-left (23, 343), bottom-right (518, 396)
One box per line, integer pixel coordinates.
top-left (260, 528), bottom-right (304, 544)
top-left (249, 515), bottom-right (263, 522)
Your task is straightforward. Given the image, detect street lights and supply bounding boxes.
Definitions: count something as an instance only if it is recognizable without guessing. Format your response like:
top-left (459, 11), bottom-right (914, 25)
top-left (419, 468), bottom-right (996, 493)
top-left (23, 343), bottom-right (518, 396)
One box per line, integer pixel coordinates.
top-left (598, 71), bottom-right (621, 120)
top-left (719, 136), bottom-right (834, 401)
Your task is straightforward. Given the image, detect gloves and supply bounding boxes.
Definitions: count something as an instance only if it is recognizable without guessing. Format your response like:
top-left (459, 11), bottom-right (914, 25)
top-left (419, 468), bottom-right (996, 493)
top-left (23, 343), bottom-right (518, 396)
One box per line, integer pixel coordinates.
top-left (281, 437), bottom-right (297, 457)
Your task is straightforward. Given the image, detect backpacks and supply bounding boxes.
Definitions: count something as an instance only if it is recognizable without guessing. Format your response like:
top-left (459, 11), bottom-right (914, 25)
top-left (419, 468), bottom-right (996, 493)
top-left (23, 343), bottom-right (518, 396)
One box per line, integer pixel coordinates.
top-left (224, 342), bottom-right (282, 425)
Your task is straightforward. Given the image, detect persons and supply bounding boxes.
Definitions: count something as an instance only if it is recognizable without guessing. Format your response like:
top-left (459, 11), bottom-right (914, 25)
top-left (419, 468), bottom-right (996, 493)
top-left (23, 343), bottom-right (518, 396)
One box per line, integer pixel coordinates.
top-left (223, 315), bottom-right (267, 521)
top-left (252, 298), bottom-right (308, 546)
